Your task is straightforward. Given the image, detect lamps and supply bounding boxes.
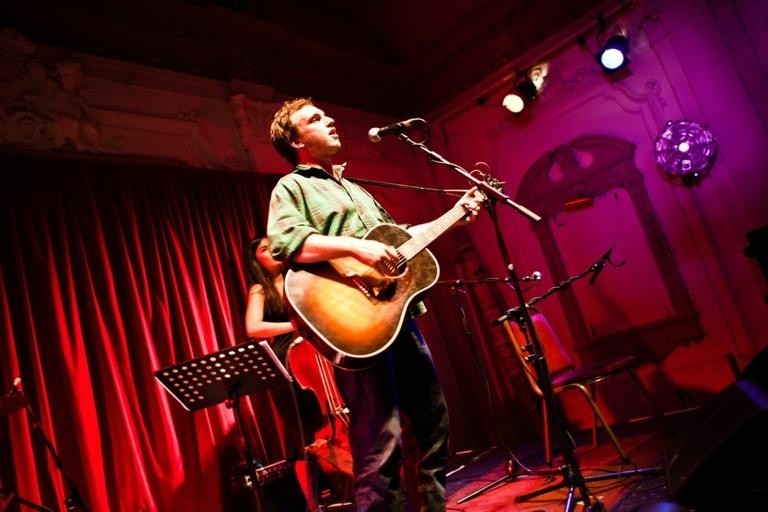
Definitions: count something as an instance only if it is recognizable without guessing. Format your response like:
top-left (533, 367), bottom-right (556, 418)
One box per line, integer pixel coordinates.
top-left (500, 68), bottom-right (536, 115)
top-left (594, 12), bottom-right (628, 71)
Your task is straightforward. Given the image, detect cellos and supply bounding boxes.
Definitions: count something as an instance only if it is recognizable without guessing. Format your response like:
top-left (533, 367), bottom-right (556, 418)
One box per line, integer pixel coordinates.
top-left (285, 333), bottom-right (355, 499)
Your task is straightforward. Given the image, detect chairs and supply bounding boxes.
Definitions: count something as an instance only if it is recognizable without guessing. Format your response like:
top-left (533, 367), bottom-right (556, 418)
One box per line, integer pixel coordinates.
top-left (504, 304), bottom-right (673, 468)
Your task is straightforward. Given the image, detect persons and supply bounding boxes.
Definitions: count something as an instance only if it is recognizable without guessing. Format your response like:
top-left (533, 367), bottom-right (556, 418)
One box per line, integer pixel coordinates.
top-left (265, 97), bottom-right (484, 511)
top-left (243, 236), bottom-right (334, 511)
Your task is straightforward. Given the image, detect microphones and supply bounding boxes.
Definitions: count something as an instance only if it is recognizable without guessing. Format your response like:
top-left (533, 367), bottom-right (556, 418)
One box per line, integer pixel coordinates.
top-left (368, 117), bottom-right (427, 145)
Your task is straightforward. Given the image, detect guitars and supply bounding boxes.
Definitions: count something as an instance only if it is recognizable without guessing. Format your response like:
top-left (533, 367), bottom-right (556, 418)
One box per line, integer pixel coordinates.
top-left (283, 175), bottom-right (502, 373)
